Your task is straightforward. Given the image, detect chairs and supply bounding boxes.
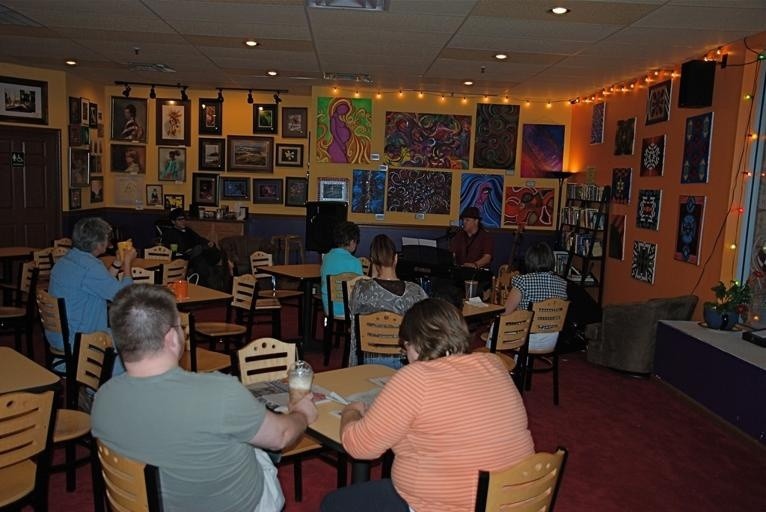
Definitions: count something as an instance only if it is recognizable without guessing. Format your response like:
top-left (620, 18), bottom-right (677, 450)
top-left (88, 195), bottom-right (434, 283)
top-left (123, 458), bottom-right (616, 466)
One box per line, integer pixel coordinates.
top-left (3, 238), bottom-right (570, 363)
top-left (526, 298), bottom-right (571, 406)
top-left (601, 295), bottom-right (699, 379)
top-left (489, 309), bottom-right (536, 397)
top-left (177, 310), bottom-right (231, 378)
top-left (88, 430), bottom-right (164, 510)
top-left (473, 446), bottom-right (569, 512)
top-left (3, 389), bottom-right (55, 511)
top-left (51, 330), bottom-right (121, 494)
top-left (354, 310), bottom-right (410, 365)
top-left (32, 287), bottom-right (82, 413)
top-left (238, 336), bottom-right (326, 502)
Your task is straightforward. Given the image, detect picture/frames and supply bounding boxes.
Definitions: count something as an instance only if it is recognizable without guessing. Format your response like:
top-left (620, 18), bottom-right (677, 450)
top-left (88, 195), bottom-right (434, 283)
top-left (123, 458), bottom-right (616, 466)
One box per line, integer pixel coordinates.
top-left (66, 95), bottom-right (309, 210)
top-left (316, 177), bottom-right (349, 204)
top-left (1, 75), bottom-right (48, 125)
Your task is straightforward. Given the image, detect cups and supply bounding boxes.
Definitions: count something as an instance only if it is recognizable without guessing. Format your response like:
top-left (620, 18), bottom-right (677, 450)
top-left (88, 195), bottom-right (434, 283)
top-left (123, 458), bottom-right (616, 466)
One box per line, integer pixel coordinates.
top-left (464, 279), bottom-right (479, 301)
top-left (174, 278), bottom-right (189, 301)
top-left (287, 360), bottom-right (314, 406)
top-left (117, 238), bottom-right (134, 263)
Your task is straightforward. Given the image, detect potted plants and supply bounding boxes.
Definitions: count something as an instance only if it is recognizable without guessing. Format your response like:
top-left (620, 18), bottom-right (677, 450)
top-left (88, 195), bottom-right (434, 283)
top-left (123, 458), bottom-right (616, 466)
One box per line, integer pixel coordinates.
top-left (704, 280), bottom-right (754, 330)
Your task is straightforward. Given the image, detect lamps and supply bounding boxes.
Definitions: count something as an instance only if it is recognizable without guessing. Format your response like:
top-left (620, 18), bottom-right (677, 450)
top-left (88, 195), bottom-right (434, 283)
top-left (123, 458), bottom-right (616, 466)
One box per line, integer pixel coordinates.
top-left (114, 81), bottom-right (289, 104)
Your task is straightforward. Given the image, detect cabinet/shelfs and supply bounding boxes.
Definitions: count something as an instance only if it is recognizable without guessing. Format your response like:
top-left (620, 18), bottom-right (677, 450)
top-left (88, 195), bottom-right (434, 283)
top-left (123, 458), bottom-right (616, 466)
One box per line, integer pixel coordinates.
top-left (653, 319), bottom-right (765, 454)
top-left (555, 183), bottom-right (613, 354)
top-left (62, 207), bottom-right (181, 255)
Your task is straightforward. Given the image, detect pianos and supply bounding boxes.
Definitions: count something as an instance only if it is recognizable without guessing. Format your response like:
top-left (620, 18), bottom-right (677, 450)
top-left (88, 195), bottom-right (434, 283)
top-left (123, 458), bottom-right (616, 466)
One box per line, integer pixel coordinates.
top-left (393, 245), bottom-right (493, 280)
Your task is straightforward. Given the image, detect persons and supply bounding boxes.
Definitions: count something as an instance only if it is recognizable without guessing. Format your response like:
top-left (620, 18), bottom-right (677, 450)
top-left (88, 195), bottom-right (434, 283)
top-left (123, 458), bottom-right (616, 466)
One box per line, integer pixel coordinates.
top-left (320, 220), bottom-right (370, 318)
top-left (45, 215), bottom-right (137, 395)
top-left (481, 240), bottom-right (568, 366)
top-left (320, 299), bottom-right (536, 512)
top-left (127, 152), bottom-right (141, 173)
top-left (449, 206), bottom-right (496, 285)
top-left (121, 105), bottom-right (138, 140)
top-left (349, 234), bottom-right (429, 369)
top-left (165, 208), bottom-right (215, 282)
top-left (89, 281), bottom-right (318, 512)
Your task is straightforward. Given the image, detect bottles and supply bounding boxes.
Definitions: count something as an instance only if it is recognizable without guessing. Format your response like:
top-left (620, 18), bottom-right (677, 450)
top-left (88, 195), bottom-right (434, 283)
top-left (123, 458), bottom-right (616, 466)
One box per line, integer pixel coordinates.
top-left (170, 244), bottom-right (178, 253)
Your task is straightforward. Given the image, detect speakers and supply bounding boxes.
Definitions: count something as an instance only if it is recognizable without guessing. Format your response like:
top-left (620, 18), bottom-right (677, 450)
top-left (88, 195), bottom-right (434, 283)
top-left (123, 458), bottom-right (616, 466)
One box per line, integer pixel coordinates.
top-left (304, 201), bottom-right (348, 253)
top-left (678, 59), bottom-right (716, 108)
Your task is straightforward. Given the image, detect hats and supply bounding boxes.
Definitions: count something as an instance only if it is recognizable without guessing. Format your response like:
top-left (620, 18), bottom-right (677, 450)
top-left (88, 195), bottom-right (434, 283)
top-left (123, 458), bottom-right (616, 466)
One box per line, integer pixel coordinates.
top-left (169, 207), bottom-right (184, 221)
top-left (459, 207), bottom-right (482, 220)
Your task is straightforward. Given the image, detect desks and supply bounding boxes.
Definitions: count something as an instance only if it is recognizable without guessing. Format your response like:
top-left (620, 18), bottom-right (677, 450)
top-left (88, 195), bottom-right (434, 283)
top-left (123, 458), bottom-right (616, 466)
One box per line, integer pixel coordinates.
top-left (2, 346), bottom-right (60, 394)
top-left (184, 216), bottom-right (244, 245)
top-left (271, 363), bottom-right (402, 486)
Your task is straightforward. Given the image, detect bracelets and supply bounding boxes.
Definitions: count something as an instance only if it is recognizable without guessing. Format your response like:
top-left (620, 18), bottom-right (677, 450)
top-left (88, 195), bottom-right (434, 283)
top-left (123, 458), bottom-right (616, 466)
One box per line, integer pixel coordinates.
top-left (111, 263), bottom-right (122, 269)
top-left (474, 262), bottom-right (480, 269)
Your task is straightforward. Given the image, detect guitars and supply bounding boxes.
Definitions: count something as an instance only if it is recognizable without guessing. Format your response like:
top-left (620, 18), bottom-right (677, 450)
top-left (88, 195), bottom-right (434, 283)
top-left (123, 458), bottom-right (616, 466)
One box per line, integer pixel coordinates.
top-left (491, 224), bottom-right (526, 307)
